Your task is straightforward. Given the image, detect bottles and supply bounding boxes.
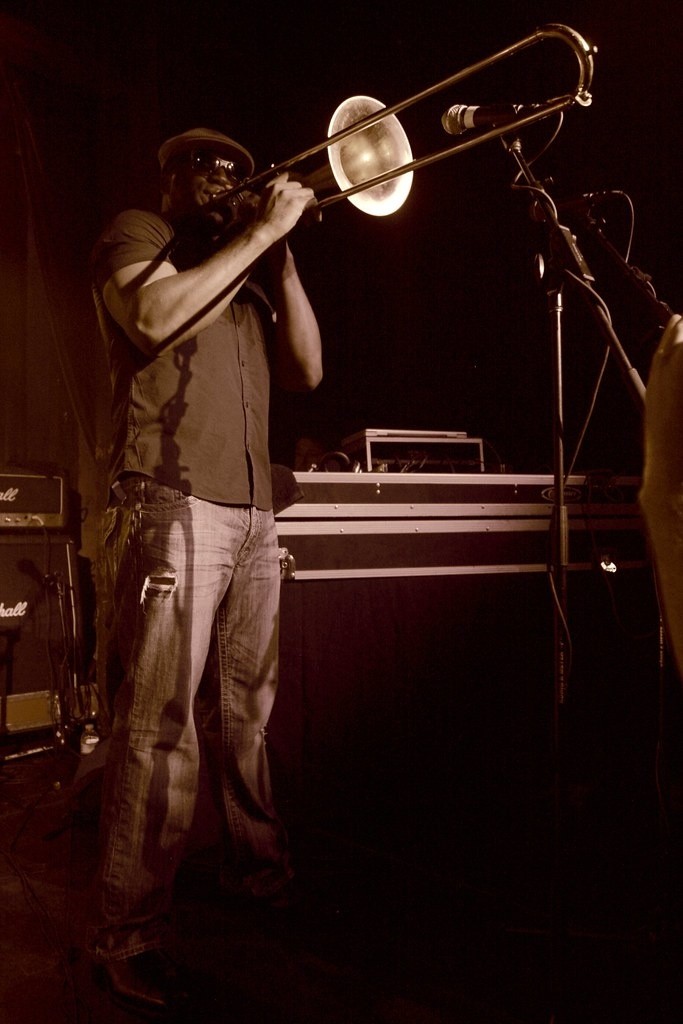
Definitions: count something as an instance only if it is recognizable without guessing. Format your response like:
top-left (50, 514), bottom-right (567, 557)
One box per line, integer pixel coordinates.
top-left (80, 724), bottom-right (100, 761)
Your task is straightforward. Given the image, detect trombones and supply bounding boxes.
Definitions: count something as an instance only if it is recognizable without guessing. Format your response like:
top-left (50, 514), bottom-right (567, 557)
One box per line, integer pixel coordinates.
top-left (188, 23), bottom-right (599, 249)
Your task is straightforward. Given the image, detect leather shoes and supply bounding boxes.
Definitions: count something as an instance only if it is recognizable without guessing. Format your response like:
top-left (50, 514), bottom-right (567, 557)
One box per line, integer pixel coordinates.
top-left (246, 881), bottom-right (343, 926)
top-left (93, 950), bottom-right (183, 1020)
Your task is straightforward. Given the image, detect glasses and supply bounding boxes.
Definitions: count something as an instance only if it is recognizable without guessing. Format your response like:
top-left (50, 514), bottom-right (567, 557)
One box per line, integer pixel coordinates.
top-left (193, 149), bottom-right (243, 182)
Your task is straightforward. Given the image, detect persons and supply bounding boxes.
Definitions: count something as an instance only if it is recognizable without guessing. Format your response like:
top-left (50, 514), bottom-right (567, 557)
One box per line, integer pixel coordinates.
top-left (61, 128), bottom-right (342, 1024)
top-left (638, 312), bottom-right (683, 680)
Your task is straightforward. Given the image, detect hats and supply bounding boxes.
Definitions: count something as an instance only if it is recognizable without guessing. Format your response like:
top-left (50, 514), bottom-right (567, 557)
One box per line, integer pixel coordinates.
top-left (158, 123), bottom-right (255, 178)
